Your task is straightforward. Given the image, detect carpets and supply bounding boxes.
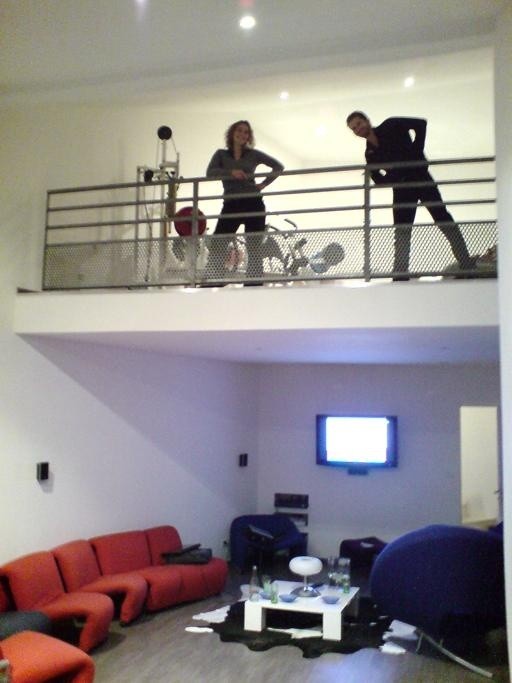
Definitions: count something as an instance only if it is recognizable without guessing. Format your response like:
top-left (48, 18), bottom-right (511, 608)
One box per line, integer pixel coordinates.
top-left (183, 594), bottom-right (419, 659)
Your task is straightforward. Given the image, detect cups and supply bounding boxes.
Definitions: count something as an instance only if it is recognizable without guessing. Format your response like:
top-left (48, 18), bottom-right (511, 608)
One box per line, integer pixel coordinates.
top-left (270, 582), bottom-right (277, 602)
top-left (344, 575), bottom-right (349, 592)
top-left (263, 575), bottom-right (270, 592)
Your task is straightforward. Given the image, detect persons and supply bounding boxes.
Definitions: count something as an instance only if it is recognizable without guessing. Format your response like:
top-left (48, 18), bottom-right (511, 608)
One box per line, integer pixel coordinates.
top-left (347, 108), bottom-right (475, 280)
top-left (195, 116), bottom-right (285, 288)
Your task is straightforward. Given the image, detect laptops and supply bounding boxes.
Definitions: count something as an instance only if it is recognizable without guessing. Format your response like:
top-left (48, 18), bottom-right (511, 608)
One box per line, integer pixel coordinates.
top-left (161, 544), bottom-right (201, 557)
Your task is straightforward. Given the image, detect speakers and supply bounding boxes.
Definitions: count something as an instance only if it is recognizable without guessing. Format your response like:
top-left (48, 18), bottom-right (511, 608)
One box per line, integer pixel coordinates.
top-left (38, 461), bottom-right (48, 481)
top-left (240, 453), bottom-right (249, 466)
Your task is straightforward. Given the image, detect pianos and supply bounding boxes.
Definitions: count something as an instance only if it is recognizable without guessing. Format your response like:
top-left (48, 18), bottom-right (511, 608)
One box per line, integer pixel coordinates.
top-left (245, 525), bottom-right (275, 543)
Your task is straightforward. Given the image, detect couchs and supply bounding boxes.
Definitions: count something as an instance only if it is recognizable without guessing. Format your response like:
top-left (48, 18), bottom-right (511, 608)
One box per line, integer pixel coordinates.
top-left (229, 512), bottom-right (309, 583)
top-left (371, 519), bottom-right (507, 680)
top-left (0, 523), bottom-right (229, 683)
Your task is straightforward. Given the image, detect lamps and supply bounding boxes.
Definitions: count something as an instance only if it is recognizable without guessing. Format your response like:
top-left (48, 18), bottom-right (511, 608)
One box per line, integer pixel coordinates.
top-left (289, 556), bottom-right (323, 598)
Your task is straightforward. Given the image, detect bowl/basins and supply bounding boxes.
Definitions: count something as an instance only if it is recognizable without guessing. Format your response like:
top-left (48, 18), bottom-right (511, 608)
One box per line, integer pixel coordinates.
top-left (322, 595), bottom-right (339, 604)
top-left (260, 591), bottom-right (277, 599)
top-left (280, 593), bottom-right (297, 602)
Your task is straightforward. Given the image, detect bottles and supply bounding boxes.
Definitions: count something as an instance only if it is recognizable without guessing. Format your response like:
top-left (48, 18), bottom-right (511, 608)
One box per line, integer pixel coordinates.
top-left (249, 565), bottom-right (259, 602)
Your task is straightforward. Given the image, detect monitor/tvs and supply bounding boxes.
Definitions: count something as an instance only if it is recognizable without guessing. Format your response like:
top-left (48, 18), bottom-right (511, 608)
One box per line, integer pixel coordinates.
top-left (316, 415), bottom-right (397, 475)
